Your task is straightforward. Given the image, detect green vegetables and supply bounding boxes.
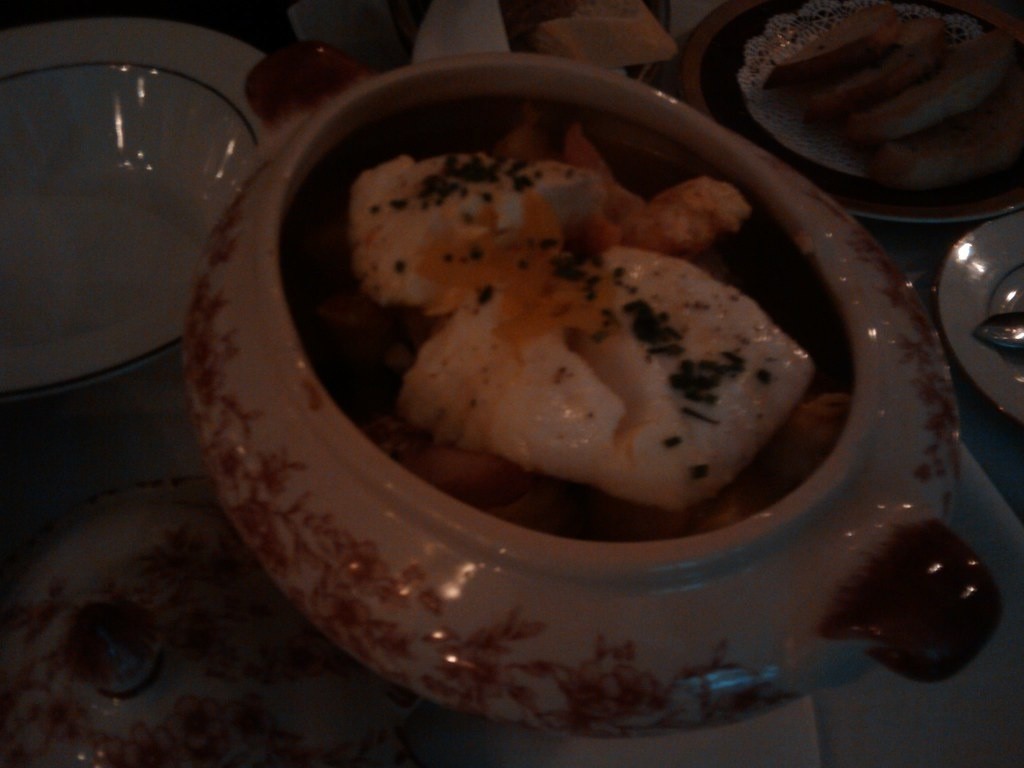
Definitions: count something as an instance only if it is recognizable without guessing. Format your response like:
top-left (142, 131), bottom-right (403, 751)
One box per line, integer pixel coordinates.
top-left (371, 149), bottom-right (775, 480)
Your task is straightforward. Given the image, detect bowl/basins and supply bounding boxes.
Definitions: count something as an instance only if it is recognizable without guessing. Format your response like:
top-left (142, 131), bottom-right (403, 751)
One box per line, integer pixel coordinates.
top-left (184, 56), bottom-right (999, 740)
top-left (391, 0), bottom-right (671, 83)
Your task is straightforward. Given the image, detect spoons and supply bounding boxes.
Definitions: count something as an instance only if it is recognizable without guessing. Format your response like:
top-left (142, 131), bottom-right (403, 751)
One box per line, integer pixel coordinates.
top-left (974, 312), bottom-right (1024, 349)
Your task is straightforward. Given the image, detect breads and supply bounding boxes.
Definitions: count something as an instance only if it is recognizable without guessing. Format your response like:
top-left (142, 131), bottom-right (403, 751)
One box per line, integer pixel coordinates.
top-left (762, 6), bottom-right (1024, 190)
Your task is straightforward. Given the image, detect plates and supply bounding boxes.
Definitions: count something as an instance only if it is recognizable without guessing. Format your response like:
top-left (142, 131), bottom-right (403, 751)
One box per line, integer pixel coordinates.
top-left (681, 0), bottom-right (1024, 221)
top-left (0, 18), bottom-right (297, 402)
top-left (932, 209), bottom-right (1024, 427)
